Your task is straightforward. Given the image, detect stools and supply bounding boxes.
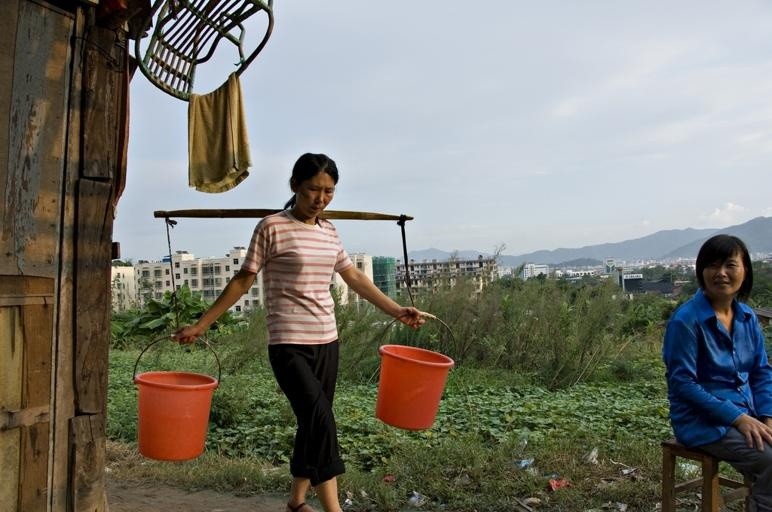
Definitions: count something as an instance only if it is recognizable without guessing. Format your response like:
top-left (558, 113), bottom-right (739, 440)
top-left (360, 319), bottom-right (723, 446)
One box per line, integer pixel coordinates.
top-left (660, 441), bottom-right (752, 512)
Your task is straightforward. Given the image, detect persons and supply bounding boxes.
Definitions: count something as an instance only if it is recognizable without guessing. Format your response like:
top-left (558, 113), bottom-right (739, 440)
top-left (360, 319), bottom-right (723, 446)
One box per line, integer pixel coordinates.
top-left (661, 234), bottom-right (771, 512)
top-left (175, 151), bottom-right (425, 512)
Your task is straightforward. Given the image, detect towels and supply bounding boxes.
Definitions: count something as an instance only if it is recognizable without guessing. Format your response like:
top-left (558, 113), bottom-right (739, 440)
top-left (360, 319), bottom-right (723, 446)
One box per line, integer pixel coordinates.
top-left (187, 71), bottom-right (253, 194)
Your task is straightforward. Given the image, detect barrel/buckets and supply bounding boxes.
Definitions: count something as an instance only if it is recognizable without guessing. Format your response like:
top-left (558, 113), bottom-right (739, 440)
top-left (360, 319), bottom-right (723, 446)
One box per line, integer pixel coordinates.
top-left (132, 334), bottom-right (221, 461)
top-left (375, 312), bottom-right (457, 430)
